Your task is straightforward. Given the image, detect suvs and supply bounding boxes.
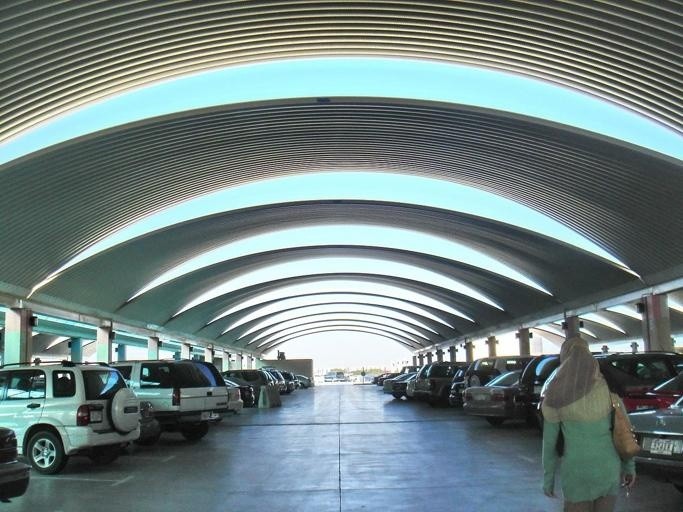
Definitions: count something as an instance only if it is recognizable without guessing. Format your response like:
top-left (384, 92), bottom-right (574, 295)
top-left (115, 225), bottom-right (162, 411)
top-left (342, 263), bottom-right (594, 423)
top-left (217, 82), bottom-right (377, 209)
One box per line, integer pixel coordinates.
top-left (109, 360), bottom-right (229, 445)
top-left (0, 358), bottom-right (141, 474)
top-left (324, 372), bottom-right (344, 382)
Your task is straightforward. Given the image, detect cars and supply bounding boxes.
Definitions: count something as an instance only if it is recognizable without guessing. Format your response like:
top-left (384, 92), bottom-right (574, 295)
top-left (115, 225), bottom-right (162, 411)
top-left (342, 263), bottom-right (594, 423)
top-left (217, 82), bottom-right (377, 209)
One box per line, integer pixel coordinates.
top-left (462, 351), bottom-right (683, 468)
top-left (220, 368), bottom-right (311, 408)
top-left (372, 361), bottom-right (469, 408)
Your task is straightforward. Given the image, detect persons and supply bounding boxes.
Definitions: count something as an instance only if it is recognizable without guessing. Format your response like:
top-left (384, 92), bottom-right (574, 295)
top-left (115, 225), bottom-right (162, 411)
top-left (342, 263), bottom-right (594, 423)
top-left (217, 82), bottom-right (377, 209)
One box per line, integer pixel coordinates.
top-left (537, 333), bottom-right (640, 512)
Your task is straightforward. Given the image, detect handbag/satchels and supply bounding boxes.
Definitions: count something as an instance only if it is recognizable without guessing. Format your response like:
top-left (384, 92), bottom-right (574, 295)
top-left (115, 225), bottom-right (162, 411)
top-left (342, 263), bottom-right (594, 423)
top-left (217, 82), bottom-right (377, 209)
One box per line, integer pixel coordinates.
top-left (609, 392), bottom-right (640, 462)
